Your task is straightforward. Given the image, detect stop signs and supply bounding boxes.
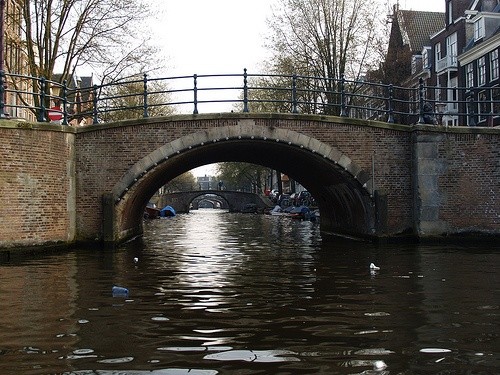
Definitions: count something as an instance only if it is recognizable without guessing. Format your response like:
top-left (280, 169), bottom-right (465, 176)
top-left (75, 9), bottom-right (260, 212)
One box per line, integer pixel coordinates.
top-left (48, 107), bottom-right (61, 120)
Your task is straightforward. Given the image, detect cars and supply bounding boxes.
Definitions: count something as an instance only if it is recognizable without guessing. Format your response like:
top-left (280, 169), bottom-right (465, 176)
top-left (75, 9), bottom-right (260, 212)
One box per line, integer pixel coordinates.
top-left (268, 189), bottom-right (319, 222)
